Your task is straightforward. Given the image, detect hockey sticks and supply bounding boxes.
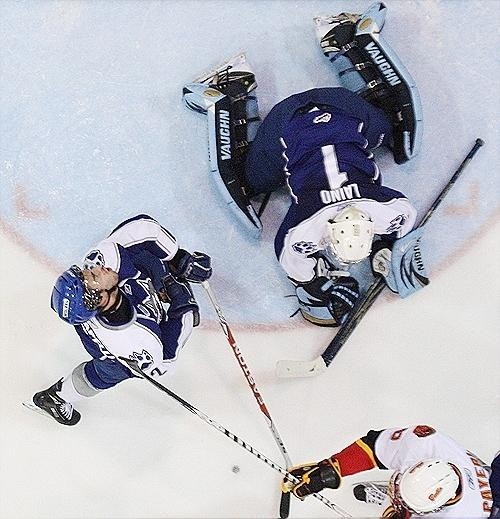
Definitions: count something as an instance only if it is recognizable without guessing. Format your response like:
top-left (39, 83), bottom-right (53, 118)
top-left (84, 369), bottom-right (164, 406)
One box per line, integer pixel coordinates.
top-left (275, 136), bottom-right (484, 379)
top-left (118, 356), bottom-right (351, 518)
top-left (201, 282), bottom-right (295, 518)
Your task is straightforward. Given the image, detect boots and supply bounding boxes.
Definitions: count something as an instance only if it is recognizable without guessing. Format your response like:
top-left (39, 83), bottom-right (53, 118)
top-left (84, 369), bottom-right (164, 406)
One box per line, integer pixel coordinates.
top-left (33, 377), bottom-right (81, 426)
top-left (353, 484), bottom-right (390, 506)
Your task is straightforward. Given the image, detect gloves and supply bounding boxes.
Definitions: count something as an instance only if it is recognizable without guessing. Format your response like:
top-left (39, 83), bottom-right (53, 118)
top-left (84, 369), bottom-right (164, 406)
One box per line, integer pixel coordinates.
top-left (177, 251), bottom-right (212, 283)
top-left (280, 457), bottom-right (341, 500)
top-left (159, 272), bottom-right (200, 328)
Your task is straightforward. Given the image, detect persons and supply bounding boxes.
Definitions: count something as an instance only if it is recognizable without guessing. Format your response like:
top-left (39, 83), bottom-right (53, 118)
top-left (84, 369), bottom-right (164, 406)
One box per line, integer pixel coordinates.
top-left (32, 214), bottom-right (215, 426)
top-left (180, 0), bottom-right (432, 329)
top-left (486, 451), bottom-right (500, 519)
top-left (280, 422), bottom-right (494, 519)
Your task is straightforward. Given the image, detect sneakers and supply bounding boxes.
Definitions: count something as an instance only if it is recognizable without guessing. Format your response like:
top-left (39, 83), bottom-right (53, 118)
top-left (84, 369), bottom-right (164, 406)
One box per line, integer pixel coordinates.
top-left (322, 19), bottom-right (362, 57)
top-left (203, 71), bottom-right (258, 102)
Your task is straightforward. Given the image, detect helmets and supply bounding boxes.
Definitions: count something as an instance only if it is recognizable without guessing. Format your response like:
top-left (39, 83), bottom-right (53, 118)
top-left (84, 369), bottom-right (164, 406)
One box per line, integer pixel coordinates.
top-left (395, 458), bottom-right (459, 517)
top-left (322, 205), bottom-right (374, 270)
top-left (50, 265), bottom-right (103, 326)
